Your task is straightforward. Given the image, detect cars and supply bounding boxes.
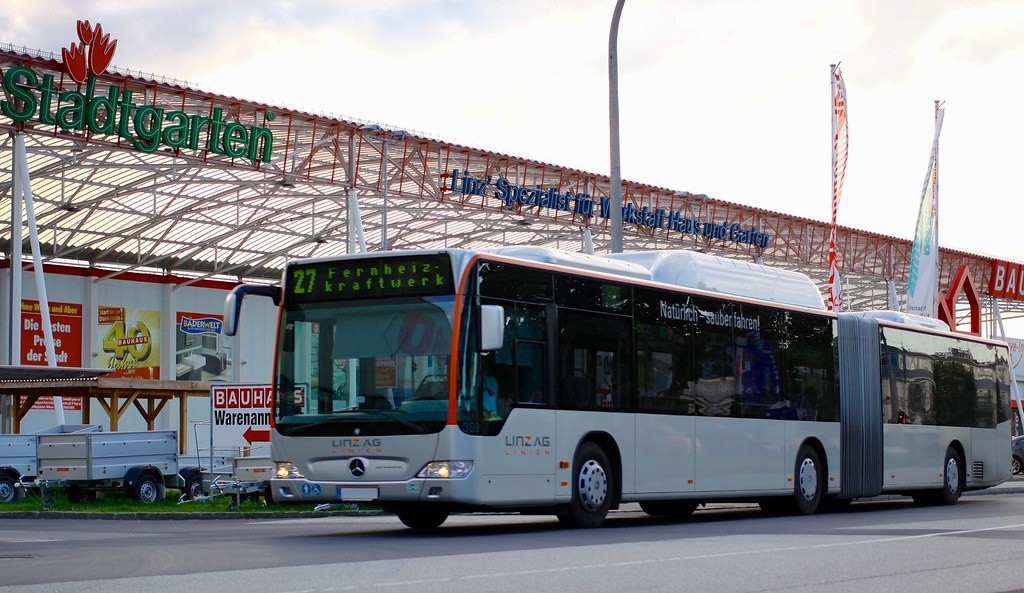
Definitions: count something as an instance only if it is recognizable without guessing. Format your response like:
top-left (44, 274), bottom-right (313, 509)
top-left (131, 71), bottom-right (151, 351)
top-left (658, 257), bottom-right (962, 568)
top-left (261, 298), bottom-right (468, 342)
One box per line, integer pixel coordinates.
top-left (1011, 436), bottom-right (1023, 475)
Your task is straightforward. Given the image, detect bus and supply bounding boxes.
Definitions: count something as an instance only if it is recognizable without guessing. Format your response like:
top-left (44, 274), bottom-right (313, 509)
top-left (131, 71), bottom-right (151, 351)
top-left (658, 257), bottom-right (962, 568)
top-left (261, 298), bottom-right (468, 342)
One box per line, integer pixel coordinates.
top-left (224, 243), bottom-right (1013, 532)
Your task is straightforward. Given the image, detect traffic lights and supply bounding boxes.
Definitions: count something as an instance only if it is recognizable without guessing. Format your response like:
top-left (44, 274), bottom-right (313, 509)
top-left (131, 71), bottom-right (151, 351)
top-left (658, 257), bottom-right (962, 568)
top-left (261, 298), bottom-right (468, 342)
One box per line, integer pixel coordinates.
top-left (897, 411), bottom-right (903, 423)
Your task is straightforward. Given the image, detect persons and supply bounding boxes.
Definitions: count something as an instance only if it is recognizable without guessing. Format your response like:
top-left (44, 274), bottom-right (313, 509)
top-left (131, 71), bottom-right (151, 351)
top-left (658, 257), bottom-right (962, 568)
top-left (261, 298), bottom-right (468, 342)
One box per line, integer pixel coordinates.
top-left (458, 358), bottom-right (498, 415)
